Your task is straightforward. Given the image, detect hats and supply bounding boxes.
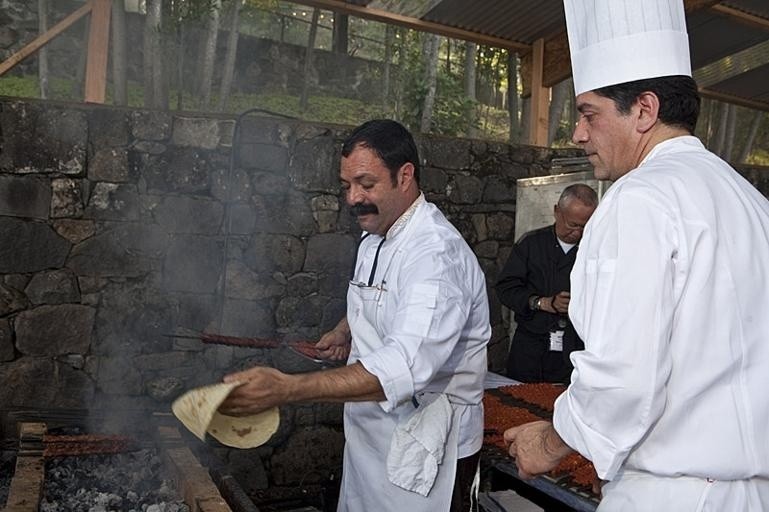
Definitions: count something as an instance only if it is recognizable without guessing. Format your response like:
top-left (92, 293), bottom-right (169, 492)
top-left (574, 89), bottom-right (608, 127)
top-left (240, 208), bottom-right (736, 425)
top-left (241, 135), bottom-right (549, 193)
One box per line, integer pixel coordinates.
top-left (562, 0), bottom-right (692, 97)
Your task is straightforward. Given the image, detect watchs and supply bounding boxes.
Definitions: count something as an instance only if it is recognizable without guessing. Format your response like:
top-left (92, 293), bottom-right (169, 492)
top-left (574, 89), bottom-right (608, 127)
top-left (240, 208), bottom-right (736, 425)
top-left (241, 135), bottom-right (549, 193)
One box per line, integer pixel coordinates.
top-left (533, 294), bottom-right (544, 311)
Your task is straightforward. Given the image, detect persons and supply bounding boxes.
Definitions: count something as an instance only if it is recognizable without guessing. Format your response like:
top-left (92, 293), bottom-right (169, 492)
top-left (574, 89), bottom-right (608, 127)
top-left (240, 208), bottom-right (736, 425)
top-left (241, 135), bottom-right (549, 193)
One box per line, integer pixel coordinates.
top-left (495, 182), bottom-right (600, 387)
top-left (216, 118), bottom-right (495, 511)
top-left (500, 0), bottom-right (769, 512)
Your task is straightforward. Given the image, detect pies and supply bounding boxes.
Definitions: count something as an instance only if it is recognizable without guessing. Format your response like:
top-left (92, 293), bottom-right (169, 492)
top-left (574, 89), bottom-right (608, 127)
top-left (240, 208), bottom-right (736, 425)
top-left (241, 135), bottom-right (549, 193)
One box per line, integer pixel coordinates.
top-left (171, 382), bottom-right (279, 450)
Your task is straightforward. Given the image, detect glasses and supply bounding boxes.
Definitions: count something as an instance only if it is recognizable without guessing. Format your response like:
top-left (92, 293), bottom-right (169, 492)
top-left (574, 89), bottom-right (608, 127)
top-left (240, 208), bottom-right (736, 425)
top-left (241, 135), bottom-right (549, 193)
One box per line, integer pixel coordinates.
top-left (559, 207), bottom-right (580, 230)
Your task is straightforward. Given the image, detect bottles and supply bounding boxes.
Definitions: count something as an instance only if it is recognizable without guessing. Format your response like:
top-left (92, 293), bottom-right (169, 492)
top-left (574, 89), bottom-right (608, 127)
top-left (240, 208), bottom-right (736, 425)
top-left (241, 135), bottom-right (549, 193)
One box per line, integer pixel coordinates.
top-left (547, 313), bottom-right (568, 354)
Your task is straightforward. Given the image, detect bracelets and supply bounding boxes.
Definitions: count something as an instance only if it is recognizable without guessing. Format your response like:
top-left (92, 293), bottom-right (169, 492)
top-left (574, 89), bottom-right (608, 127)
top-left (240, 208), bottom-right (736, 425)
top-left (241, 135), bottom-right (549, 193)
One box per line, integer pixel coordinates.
top-left (551, 295), bottom-right (559, 313)
